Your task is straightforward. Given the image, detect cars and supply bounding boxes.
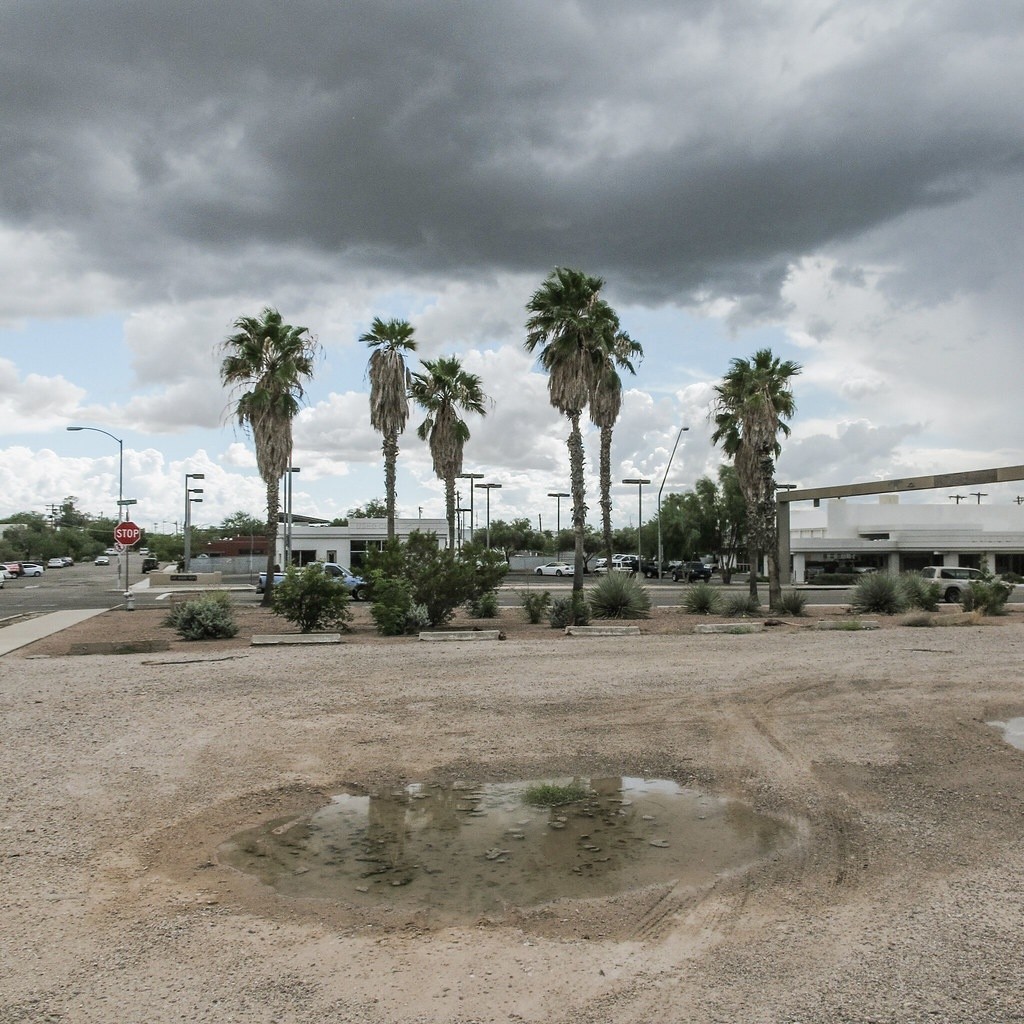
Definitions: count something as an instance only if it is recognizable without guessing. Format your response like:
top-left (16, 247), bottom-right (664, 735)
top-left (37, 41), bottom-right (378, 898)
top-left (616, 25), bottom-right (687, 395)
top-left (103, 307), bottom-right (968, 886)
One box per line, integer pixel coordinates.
top-left (94, 555), bottom-right (109, 566)
top-left (140, 558), bottom-right (159, 573)
top-left (593, 552), bottom-right (640, 576)
top-left (22, 563), bottom-right (44, 576)
top-left (47, 556), bottom-right (73, 569)
top-left (139, 548), bottom-right (150, 555)
top-left (534, 561), bottom-right (575, 577)
top-left (1, 563), bottom-right (21, 587)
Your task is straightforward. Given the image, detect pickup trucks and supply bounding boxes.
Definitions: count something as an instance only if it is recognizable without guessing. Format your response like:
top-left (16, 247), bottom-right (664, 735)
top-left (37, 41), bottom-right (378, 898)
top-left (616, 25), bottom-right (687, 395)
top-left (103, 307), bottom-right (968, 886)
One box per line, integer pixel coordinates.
top-left (671, 562), bottom-right (712, 584)
top-left (256, 561), bottom-right (370, 601)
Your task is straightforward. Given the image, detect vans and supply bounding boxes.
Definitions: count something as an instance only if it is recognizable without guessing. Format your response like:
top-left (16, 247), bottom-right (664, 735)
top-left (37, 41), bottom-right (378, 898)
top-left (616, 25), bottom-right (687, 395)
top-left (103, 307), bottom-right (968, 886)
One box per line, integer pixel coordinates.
top-left (919, 566), bottom-right (1011, 602)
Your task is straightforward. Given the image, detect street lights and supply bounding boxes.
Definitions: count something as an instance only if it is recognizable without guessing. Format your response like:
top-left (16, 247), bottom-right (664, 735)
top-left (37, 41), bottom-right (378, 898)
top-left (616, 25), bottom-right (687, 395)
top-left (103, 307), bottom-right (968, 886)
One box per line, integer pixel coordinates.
top-left (66, 425), bottom-right (123, 589)
top-left (657, 426), bottom-right (690, 581)
top-left (455, 472), bottom-right (485, 540)
top-left (622, 478), bottom-right (651, 573)
top-left (547, 492), bottom-right (570, 561)
top-left (474, 481), bottom-right (503, 551)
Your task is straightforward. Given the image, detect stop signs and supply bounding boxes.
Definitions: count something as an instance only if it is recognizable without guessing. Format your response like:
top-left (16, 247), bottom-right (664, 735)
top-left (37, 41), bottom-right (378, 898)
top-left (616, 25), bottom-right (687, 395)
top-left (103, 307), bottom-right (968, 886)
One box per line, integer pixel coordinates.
top-left (114, 521), bottom-right (142, 545)
